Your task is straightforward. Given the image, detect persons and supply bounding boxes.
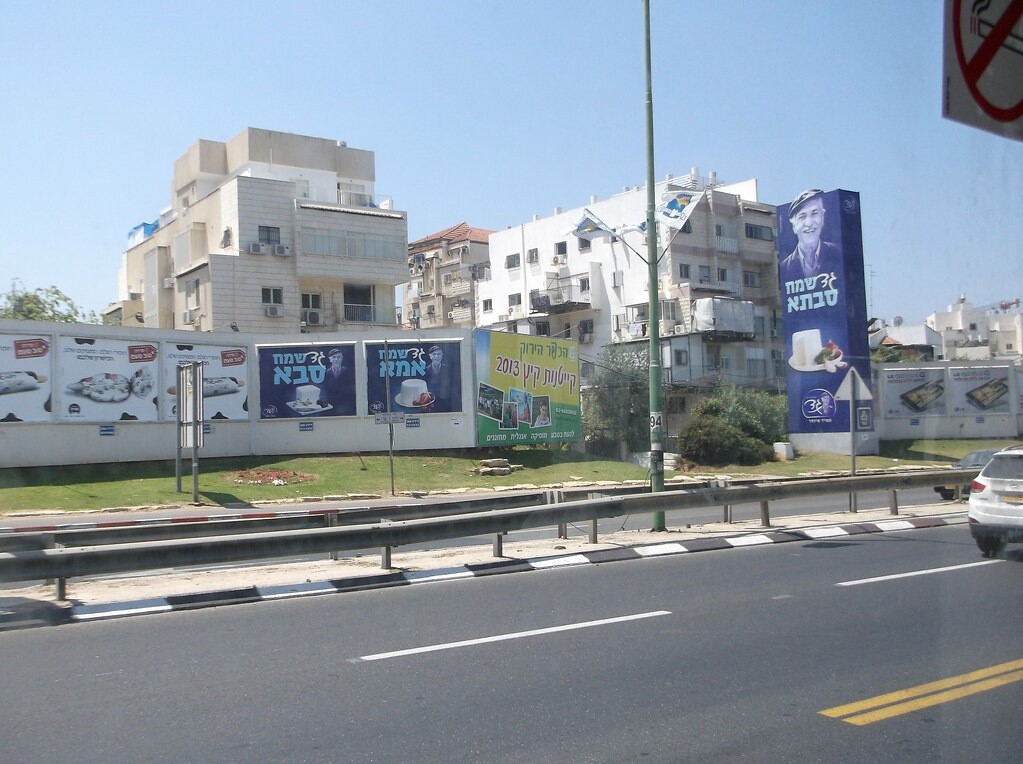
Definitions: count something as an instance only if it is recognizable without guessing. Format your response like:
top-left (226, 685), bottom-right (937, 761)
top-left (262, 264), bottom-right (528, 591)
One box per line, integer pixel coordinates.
top-left (534, 400), bottom-right (549, 426)
top-left (779, 188), bottom-right (837, 281)
top-left (324, 348), bottom-right (347, 382)
top-left (426, 345), bottom-right (448, 375)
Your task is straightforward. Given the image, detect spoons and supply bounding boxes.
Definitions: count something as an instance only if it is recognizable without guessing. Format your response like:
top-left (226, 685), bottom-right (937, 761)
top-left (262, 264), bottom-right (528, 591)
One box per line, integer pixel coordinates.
top-left (823, 358), bottom-right (837, 373)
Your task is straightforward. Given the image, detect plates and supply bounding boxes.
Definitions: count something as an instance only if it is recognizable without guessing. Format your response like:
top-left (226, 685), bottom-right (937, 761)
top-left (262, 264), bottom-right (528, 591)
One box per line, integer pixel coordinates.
top-left (395, 391), bottom-right (435, 407)
top-left (788, 348), bottom-right (842, 372)
top-left (287, 400), bottom-right (333, 415)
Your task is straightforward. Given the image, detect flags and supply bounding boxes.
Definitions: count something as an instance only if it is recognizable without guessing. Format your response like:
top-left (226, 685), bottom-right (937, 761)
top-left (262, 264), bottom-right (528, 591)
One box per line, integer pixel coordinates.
top-left (564, 208), bottom-right (614, 243)
top-left (653, 189), bottom-right (705, 231)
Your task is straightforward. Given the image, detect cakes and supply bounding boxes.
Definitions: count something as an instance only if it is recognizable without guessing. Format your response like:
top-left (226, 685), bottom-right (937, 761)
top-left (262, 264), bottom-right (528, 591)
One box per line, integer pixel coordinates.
top-left (400, 379), bottom-right (430, 405)
top-left (292, 385), bottom-right (322, 410)
top-left (792, 329), bottom-right (823, 366)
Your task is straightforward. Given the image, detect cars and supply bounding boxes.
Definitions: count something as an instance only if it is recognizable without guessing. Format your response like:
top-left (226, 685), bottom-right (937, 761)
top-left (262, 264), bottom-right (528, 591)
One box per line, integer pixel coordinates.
top-left (934, 449), bottom-right (1003, 501)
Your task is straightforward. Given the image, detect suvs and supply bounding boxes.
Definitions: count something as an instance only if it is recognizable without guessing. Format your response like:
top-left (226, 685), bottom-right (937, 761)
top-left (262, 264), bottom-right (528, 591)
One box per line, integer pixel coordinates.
top-left (968, 443), bottom-right (1023, 552)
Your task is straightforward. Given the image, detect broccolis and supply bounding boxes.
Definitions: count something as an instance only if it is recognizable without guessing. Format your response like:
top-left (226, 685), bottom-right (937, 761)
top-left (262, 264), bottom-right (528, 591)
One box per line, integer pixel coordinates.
top-left (815, 348), bottom-right (835, 364)
